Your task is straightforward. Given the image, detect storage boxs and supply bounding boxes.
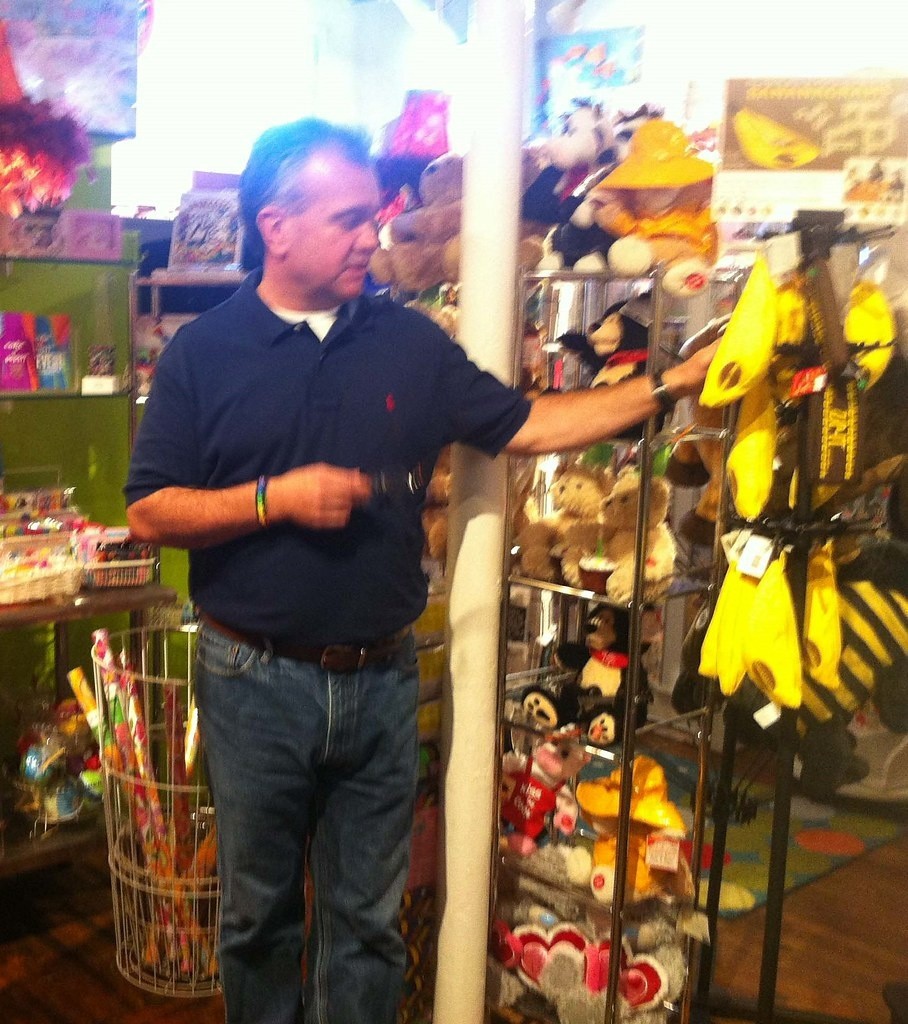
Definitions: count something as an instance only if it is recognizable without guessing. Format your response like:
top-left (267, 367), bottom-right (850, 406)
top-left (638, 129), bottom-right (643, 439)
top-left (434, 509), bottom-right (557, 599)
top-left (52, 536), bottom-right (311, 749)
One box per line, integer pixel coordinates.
top-left (167, 171), bottom-right (247, 273)
top-left (0, 0), bottom-right (138, 141)
top-left (81, 374), bottom-right (119, 395)
top-left (59, 209), bottom-right (122, 261)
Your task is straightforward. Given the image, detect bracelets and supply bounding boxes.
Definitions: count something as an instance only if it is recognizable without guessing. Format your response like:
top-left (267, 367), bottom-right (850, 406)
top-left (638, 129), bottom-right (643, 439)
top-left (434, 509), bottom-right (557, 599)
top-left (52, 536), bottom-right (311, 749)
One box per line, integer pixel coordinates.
top-left (255, 474), bottom-right (268, 528)
top-left (652, 367), bottom-right (676, 409)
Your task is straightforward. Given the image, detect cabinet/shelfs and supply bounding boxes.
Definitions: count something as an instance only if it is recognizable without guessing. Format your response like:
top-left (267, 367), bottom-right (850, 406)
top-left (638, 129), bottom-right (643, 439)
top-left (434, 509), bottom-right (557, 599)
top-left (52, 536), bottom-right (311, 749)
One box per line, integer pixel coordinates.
top-left (482, 266), bottom-right (908, 1024)
top-left (0, 120), bottom-right (250, 878)
top-left (419, 469), bottom-right (456, 746)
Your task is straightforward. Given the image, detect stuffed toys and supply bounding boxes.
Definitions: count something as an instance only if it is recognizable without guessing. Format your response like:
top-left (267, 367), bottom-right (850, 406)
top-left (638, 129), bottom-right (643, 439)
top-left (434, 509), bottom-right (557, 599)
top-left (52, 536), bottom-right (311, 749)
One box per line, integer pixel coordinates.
top-left (497, 730), bottom-right (695, 909)
top-left (520, 599), bottom-right (655, 743)
top-left (486, 872), bottom-right (690, 1024)
top-left (402, 290), bottom-right (680, 610)
top-left (366, 107), bottom-right (726, 298)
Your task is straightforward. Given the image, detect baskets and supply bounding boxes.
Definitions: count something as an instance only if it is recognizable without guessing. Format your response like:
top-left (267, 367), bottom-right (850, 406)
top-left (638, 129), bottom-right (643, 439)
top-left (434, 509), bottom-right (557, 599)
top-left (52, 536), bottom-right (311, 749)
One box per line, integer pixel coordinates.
top-left (84, 558), bottom-right (156, 588)
top-left (0, 489), bottom-right (80, 607)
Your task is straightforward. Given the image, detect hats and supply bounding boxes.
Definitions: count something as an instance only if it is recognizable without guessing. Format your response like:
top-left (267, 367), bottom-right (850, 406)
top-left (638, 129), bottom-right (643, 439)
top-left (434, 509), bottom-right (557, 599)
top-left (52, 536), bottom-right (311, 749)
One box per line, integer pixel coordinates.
top-left (597, 121), bottom-right (715, 189)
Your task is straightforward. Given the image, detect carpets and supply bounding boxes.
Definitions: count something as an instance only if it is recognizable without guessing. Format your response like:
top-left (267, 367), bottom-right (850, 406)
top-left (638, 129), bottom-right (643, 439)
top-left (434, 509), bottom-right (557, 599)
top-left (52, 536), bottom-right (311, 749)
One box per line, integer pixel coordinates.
top-left (573, 744), bottom-right (902, 916)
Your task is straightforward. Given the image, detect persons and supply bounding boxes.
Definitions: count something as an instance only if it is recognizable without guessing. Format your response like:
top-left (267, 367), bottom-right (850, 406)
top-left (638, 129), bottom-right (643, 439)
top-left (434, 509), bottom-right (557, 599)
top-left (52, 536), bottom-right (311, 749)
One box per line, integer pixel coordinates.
top-left (121, 113), bottom-right (721, 1024)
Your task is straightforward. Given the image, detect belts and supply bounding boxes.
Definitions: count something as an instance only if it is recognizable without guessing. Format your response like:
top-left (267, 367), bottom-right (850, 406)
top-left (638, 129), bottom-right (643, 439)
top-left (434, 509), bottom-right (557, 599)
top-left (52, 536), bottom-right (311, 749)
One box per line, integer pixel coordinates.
top-left (197, 610), bottom-right (410, 672)
